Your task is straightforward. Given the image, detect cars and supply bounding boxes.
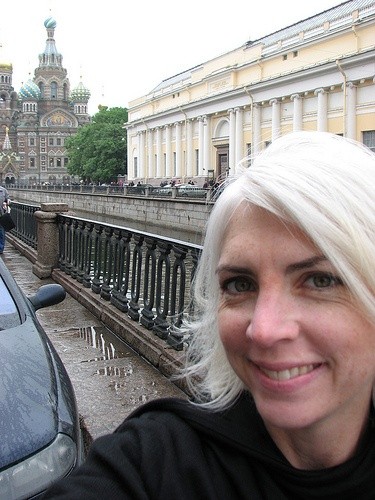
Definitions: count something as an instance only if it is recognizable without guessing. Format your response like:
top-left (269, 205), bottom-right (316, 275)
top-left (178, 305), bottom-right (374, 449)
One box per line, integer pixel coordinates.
top-left (129, 183), bottom-right (207, 198)
top-left (0, 256), bottom-right (86, 500)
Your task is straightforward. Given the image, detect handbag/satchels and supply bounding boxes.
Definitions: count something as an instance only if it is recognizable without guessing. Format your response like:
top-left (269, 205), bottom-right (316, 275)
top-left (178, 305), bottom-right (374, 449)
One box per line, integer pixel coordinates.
top-left (0, 213), bottom-right (16, 232)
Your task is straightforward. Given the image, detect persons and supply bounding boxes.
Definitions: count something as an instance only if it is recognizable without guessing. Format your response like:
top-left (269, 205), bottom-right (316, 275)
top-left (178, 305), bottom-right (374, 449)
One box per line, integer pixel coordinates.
top-left (30, 127), bottom-right (375, 500)
top-left (32, 178), bottom-right (220, 190)
top-left (0, 186), bottom-right (10, 254)
